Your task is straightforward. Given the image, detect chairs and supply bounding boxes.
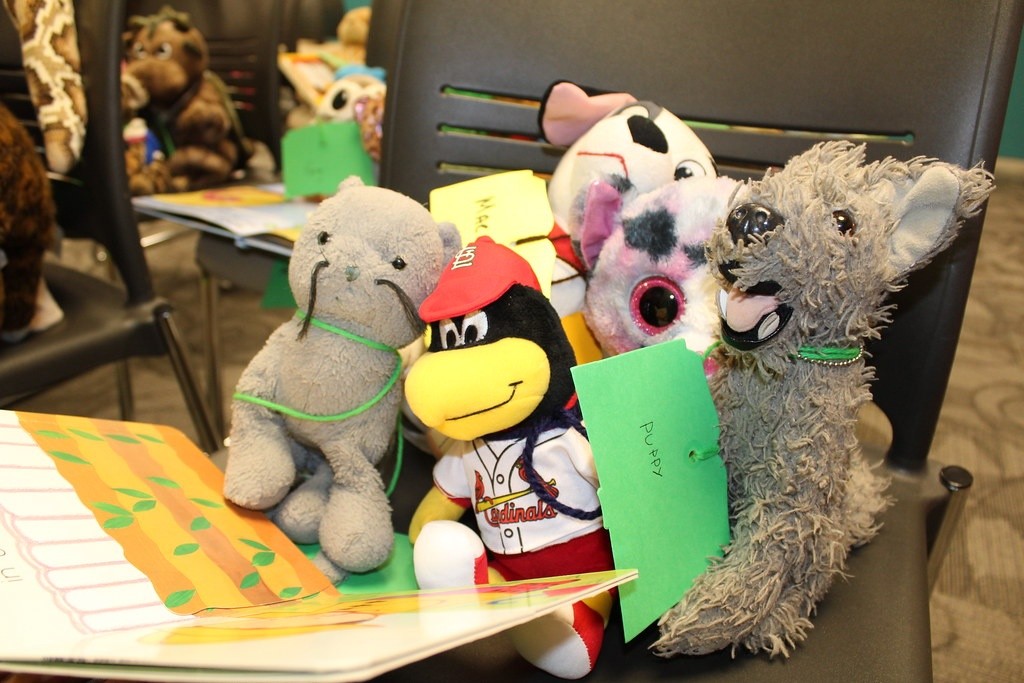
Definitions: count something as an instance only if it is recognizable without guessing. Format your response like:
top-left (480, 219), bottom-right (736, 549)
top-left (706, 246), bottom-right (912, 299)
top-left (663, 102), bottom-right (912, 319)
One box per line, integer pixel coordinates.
top-left (0, 0), bottom-right (1024, 683)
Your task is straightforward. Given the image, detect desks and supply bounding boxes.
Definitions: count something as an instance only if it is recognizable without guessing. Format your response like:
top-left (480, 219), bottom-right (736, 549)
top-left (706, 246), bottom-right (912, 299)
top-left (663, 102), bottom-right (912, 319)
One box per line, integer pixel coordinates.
top-left (131, 182), bottom-right (328, 448)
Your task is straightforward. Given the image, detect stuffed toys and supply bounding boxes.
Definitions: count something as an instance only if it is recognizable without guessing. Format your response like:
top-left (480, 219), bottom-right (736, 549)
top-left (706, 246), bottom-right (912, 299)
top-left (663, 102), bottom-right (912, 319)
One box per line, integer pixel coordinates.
top-left (4, 0), bottom-right (88, 174)
top-left (537, 82), bottom-right (754, 378)
top-left (0, 105), bottom-right (65, 342)
top-left (223, 175), bottom-right (463, 572)
top-left (648, 140), bottom-right (997, 660)
top-left (121, 5), bottom-right (256, 191)
top-left (404, 235), bottom-right (623, 679)
top-left (277, 5), bottom-right (387, 203)
